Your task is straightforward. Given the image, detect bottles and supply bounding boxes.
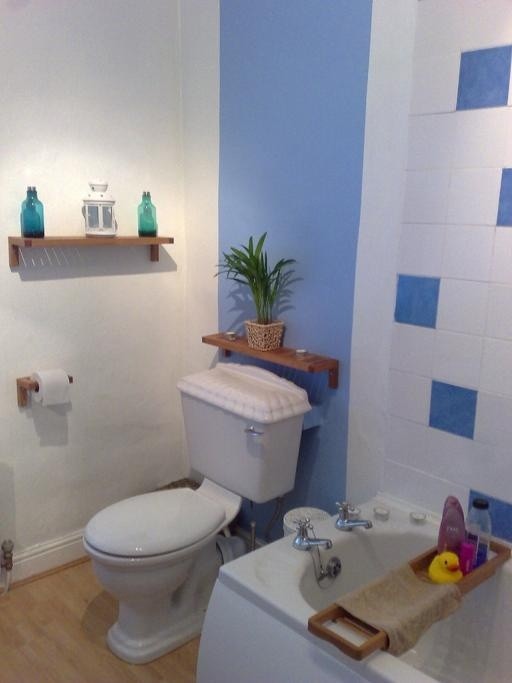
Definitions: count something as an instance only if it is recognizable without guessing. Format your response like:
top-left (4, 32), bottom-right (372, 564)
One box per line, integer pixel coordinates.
top-left (137, 189), bottom-right (156, 236)
top-left (19, 186), bottom-right (43, 238)
top-left (460, 498), bottom-right (491, 575)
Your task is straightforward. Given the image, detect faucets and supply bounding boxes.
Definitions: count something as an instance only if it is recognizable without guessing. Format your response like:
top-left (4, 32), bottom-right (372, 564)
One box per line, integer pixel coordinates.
top-left (337, 500), bottom-right (372, 532)
top-left (292, 512), bottom-right (331, 551)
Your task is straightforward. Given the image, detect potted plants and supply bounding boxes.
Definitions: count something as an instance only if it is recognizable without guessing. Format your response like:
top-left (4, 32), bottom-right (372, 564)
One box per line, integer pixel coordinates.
top-left (217, 231), bottom-right (304, 350)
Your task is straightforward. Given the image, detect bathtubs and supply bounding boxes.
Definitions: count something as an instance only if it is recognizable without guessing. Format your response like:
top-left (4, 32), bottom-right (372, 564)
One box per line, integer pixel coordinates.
top-left (196, 498), bottom-right (512, 682)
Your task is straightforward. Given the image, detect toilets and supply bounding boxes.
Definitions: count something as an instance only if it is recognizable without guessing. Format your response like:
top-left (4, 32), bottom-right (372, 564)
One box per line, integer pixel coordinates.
top-left (81, 361), bottom-right (309, 664)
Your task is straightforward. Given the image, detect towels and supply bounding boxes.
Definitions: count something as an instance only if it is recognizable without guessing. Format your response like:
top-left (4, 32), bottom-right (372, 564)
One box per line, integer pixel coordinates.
top-left (334, 565), bottom-right (462, 659)
